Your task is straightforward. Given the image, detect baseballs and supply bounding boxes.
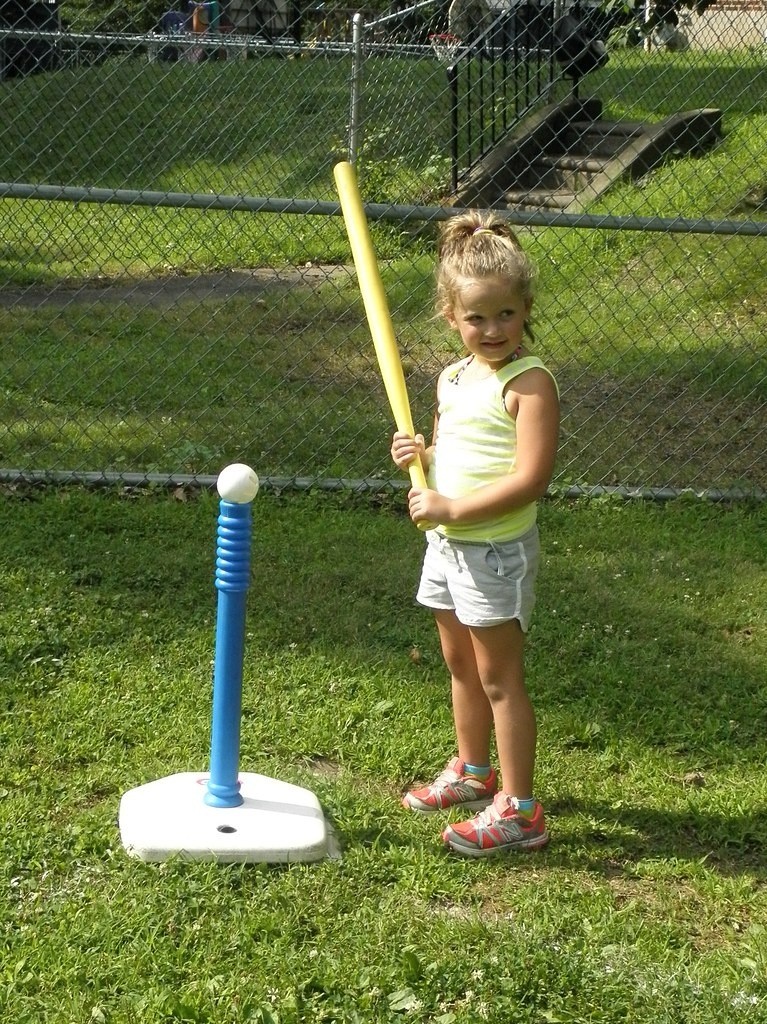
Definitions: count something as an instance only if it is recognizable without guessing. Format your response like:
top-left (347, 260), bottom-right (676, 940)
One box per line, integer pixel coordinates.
top-left (216, 463), bottom-right (259, 504)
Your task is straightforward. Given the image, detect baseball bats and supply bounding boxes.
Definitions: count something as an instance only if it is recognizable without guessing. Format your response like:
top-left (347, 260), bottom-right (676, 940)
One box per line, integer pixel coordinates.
top-left (333, 160), bottom-right (440, 532)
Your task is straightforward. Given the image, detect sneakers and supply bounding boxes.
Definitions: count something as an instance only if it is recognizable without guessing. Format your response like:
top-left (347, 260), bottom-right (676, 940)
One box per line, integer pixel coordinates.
top-left (442, 791), bottom-right (549, 857)
top-left (402, 756), bottom-right (499, 815)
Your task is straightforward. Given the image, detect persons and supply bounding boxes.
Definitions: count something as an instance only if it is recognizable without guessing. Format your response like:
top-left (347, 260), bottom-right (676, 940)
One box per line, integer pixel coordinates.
top-left (390, 207), bottom-right (562, 855)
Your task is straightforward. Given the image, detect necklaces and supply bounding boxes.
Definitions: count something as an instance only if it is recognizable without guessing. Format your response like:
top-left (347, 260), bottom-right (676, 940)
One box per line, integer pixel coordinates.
top-left (448, 344), bottom-right (523, 413)
